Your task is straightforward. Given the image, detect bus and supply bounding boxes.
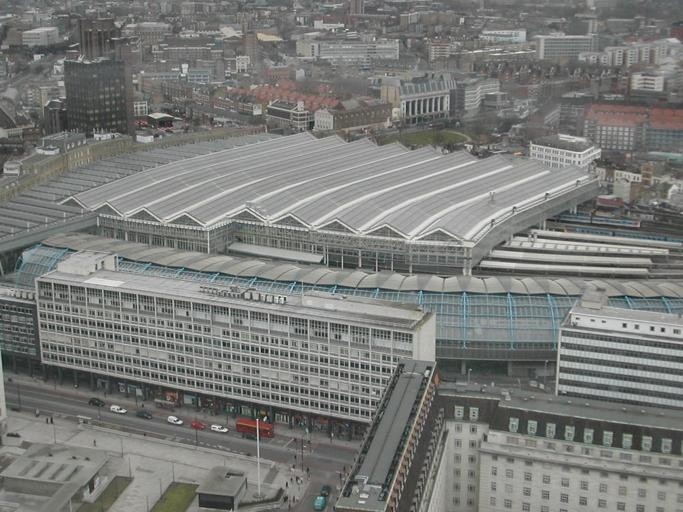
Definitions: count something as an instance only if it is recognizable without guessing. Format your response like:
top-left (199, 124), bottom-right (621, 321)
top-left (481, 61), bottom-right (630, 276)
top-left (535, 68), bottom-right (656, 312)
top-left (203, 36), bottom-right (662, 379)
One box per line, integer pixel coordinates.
top-left (236, 417), bottom-right (274, 439)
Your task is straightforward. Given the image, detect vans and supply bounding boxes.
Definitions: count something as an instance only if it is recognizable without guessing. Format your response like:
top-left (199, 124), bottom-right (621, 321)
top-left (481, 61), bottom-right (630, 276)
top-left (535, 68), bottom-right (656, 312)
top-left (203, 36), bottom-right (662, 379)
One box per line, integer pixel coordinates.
top-left (167, 415), bottom-right (184, 426)
top-left (210, 424), bottom-right (229, 433)
top-left (111, 405), bottom-right (127, 414)
top-left (313, 496), bottom-right (327, 511)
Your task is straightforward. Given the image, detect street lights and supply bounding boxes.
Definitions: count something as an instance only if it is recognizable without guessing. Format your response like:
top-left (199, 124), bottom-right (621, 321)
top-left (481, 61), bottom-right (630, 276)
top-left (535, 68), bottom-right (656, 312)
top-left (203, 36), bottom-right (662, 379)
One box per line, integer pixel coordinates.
top-left (256, 416), bottom-right (268, 496)
top-left (467, 368), bottom-right (472, 383)
top-left (543, 360), bottom-right (549, 392)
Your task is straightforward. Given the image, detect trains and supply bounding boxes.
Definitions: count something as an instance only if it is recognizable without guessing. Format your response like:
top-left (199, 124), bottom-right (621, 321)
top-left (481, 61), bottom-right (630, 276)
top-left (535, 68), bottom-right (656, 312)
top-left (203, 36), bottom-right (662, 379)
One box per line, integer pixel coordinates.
top-left (545, 220), bottom-right (682, 245)
top-left (546, 211), bottom-right (642, 230)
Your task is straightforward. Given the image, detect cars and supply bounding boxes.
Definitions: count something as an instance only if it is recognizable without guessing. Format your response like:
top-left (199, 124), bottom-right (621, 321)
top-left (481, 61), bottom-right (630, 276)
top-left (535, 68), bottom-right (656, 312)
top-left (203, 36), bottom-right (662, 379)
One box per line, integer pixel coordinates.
top-left (136, 410), bottom-right (153, 420)
top-left (320, 485), bottom-right (331, 497)
top-left (191, 420), bottom-right (208, 431)
top-left (88, 398), bottom-right (104, 407)
top-left (242, 433), bottom-right (261, 441)
